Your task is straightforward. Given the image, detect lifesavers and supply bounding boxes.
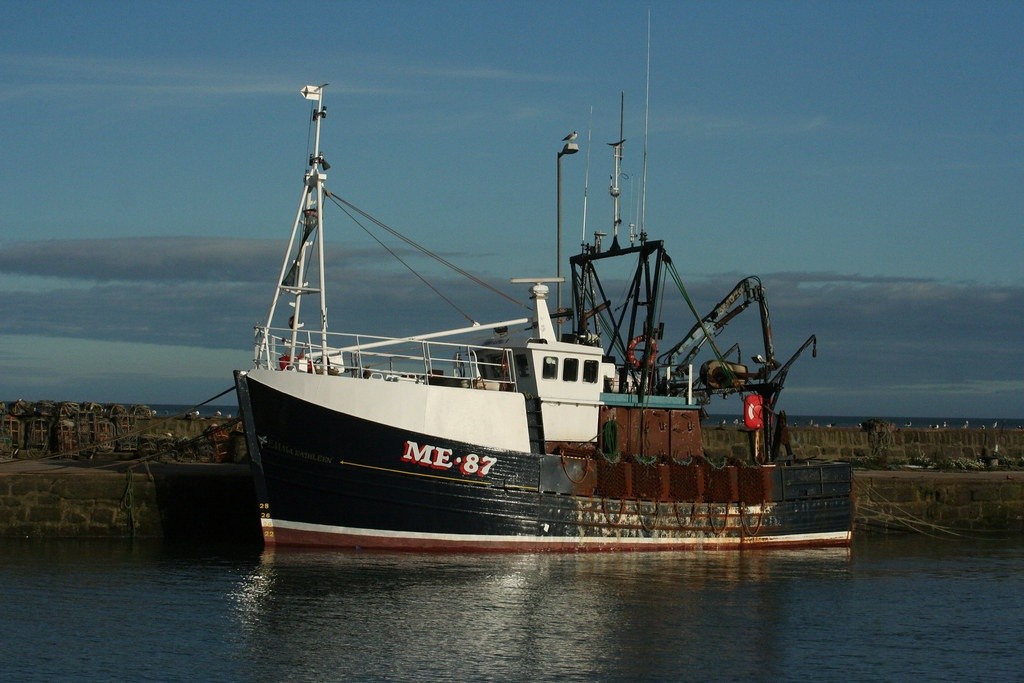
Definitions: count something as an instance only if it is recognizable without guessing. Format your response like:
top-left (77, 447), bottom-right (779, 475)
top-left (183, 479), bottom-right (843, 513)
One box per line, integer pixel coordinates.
top-left (627, 335), bottom-right (657, 366)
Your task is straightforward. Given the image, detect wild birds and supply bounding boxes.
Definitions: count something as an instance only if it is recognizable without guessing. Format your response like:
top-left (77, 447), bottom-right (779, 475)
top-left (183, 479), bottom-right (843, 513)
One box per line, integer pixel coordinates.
top-left (151, 409), bottom-right (233, 419)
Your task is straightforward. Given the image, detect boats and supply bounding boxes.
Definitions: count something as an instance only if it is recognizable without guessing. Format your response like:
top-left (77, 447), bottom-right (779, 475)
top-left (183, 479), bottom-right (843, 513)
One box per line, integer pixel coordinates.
top-left (229, 5), bottom-right (856, 553)
